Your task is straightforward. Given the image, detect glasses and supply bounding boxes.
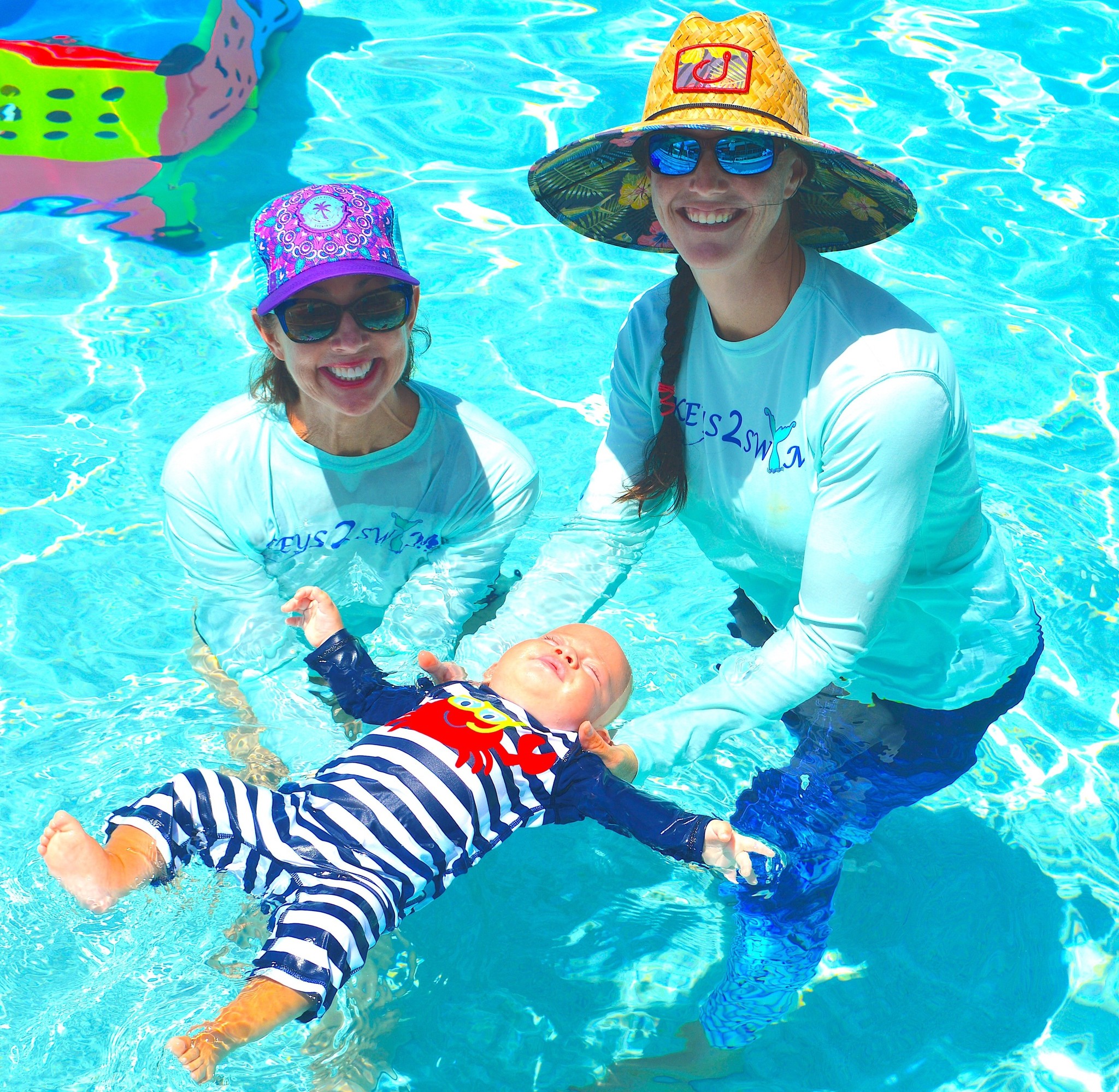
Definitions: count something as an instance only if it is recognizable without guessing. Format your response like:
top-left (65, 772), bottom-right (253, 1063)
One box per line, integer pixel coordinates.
top-left (647, 134), bottom-right (788, 175)
top-left (267, 285), bottom-right (415, 344)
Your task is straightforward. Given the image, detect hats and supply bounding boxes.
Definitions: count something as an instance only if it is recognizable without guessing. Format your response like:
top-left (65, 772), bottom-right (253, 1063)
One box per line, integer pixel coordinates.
top-left (528, 10), bottom-right (918, 255)
top-left (249, 183), bottom-right (420, 315)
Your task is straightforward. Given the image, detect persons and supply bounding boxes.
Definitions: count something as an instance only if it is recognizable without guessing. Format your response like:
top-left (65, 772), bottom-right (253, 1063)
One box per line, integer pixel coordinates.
top-left (415, 14), bottom-right (1050, 1057)
top-left (163, 185), bottom-right (546, 781)
top-left (36, 583), bottom-right (780, 1085)
